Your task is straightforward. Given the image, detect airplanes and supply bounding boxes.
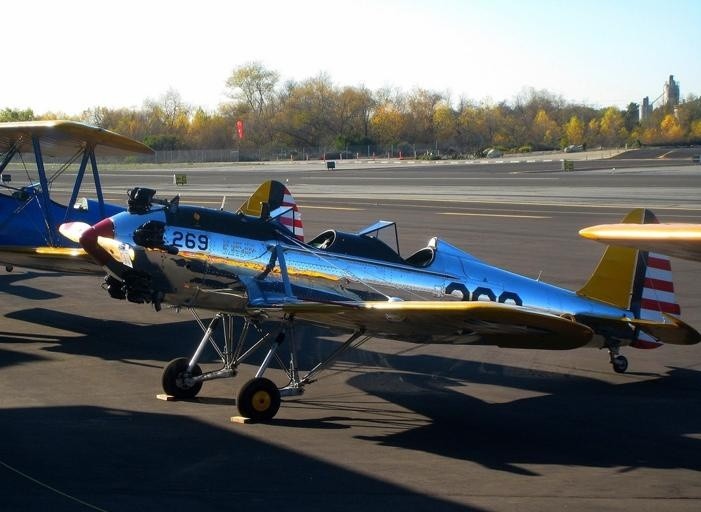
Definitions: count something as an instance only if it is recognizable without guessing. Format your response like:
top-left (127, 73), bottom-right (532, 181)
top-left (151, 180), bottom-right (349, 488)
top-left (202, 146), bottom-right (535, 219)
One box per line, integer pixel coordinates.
top-left (76, 120), bottom-right (701, 422)
top-left (578, 222), bottom-right (701, 265)
top-left (0, 119), bottom-right (305, 277)
